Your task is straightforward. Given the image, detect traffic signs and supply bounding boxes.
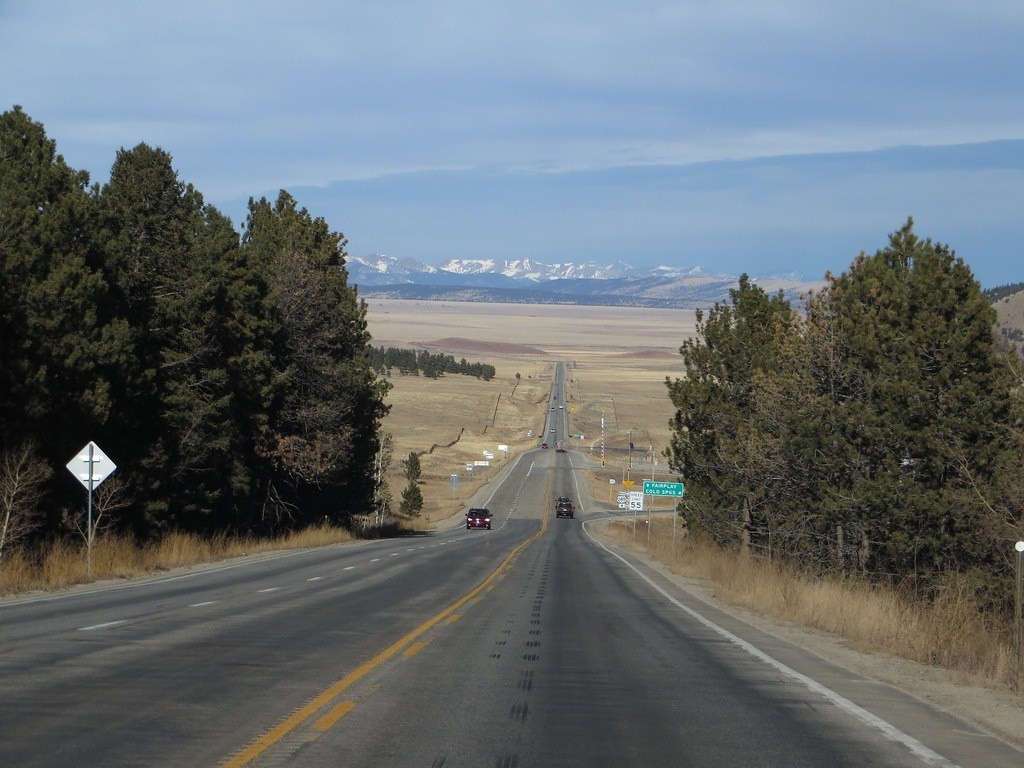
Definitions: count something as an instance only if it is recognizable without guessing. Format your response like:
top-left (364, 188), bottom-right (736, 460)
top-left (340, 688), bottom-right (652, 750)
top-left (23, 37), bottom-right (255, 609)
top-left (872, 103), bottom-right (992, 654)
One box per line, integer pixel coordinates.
top-left (642, 481), bottom-right (684, 498)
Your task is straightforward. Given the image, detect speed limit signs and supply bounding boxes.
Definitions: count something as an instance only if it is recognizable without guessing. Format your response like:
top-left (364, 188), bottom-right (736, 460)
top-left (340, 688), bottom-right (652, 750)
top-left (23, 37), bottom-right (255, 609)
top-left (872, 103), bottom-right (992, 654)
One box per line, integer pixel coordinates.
top-left (630, 492), bottom-right (643, 511)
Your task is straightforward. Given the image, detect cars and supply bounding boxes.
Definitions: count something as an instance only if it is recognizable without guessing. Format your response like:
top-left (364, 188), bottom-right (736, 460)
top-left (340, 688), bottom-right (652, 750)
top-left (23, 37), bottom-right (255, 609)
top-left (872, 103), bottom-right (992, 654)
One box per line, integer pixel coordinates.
top-left (551, 408), bottom-right (555, 412)
top-left (541, 443), bottom-right (548, 449)
top-left (550, 428), bottom-right (556, 433)
top-left (555, 497), bottom-right (573, 503)
top-left (555, 441), bottom-right (568, 453)
top-left (555, 501), bottom-right (575, 518)
top-left (559, 404), bottom-right (564, 409)
top-left (465, 508), bottom-right (493, 530)
